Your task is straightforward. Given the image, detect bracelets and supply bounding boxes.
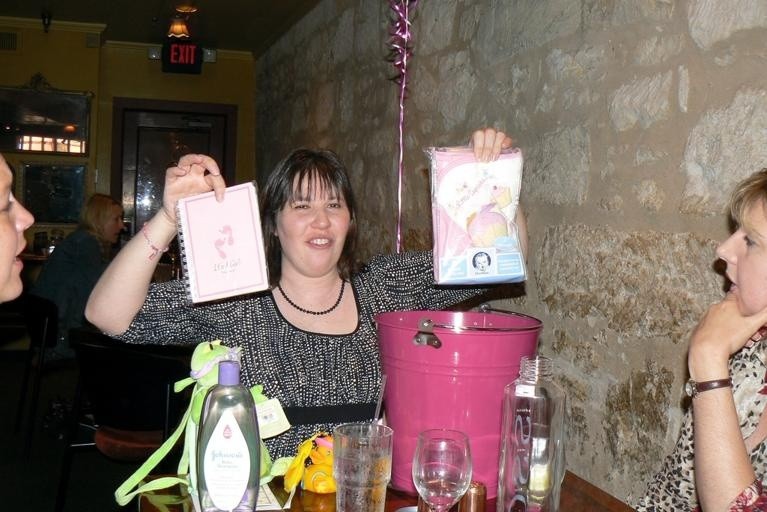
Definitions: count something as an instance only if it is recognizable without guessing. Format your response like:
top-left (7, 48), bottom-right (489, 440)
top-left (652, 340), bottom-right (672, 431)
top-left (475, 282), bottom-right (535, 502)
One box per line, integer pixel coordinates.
top-left (142, 218), bottom-right (169, 264)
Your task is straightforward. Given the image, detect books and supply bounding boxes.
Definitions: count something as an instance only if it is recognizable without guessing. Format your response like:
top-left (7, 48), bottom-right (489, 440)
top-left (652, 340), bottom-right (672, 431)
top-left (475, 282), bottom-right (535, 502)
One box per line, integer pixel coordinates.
top-left (172, 181), bottom-right (270, 306)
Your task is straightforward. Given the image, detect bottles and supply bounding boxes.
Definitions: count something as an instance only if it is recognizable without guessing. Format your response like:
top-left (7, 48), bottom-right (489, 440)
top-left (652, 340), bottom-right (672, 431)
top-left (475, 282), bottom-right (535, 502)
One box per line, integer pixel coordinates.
top-left (496, 351), bottom-right (565, 511)
top-left (195, 360), bottom-right (261, 512)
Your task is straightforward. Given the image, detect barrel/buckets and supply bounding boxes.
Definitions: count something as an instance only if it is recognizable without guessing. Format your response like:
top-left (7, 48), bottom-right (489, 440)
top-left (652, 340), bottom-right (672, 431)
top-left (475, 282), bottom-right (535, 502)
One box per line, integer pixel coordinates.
top-left (377, 310), bottom-right (542, 501)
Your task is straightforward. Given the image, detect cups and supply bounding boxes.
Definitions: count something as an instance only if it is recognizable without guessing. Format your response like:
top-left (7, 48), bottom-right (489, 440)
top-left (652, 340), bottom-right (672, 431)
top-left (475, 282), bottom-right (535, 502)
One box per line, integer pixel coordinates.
top-left (331, 421), bottom-right (393, 511)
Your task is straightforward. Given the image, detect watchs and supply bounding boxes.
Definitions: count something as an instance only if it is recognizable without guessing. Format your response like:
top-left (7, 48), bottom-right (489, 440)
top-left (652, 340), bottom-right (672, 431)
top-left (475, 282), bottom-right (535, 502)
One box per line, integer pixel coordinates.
top-left (682, 378), bottom-right (732, 397)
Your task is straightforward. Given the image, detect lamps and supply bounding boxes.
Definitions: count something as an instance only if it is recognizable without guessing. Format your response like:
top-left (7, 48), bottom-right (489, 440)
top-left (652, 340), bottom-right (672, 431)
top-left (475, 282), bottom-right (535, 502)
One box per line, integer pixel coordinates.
top-left (166, 16), bottom-right (190, 39)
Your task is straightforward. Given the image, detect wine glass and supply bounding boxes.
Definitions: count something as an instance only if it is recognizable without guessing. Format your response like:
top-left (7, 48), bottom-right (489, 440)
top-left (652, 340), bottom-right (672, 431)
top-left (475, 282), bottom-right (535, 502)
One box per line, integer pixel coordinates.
top-left (409, 425), bottom-right (476, 512)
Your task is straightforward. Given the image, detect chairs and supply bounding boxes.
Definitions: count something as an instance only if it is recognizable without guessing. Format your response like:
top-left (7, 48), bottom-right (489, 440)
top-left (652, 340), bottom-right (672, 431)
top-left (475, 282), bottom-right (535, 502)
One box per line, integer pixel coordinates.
top-left (14, 292), bottom-right (192, 511)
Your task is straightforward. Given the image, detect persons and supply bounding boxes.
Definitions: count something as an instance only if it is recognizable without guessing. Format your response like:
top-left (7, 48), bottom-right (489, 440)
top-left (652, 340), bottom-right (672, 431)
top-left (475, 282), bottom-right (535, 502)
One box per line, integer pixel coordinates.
top-left (83, 127), bottom-right (530, 482)
top-left (0, 151), bottom-right (37, 305)
top-left (636, 168), bottom-right (767, 512)
top-left (28, 193), bottom-right (126, 366)
top-left (473, 252), bottom-right (491, 275)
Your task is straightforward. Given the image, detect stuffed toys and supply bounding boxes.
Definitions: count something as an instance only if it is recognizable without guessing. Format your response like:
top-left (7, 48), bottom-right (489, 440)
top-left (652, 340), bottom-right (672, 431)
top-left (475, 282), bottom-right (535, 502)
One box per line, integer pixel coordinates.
top-left (283, 430), bottom-right (348, 494)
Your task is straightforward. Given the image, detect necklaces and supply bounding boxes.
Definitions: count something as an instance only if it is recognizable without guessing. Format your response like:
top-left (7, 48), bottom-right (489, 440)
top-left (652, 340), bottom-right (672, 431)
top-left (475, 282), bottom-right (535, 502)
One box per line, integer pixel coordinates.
top-left (276, 279), bottom-right (346, 315)
